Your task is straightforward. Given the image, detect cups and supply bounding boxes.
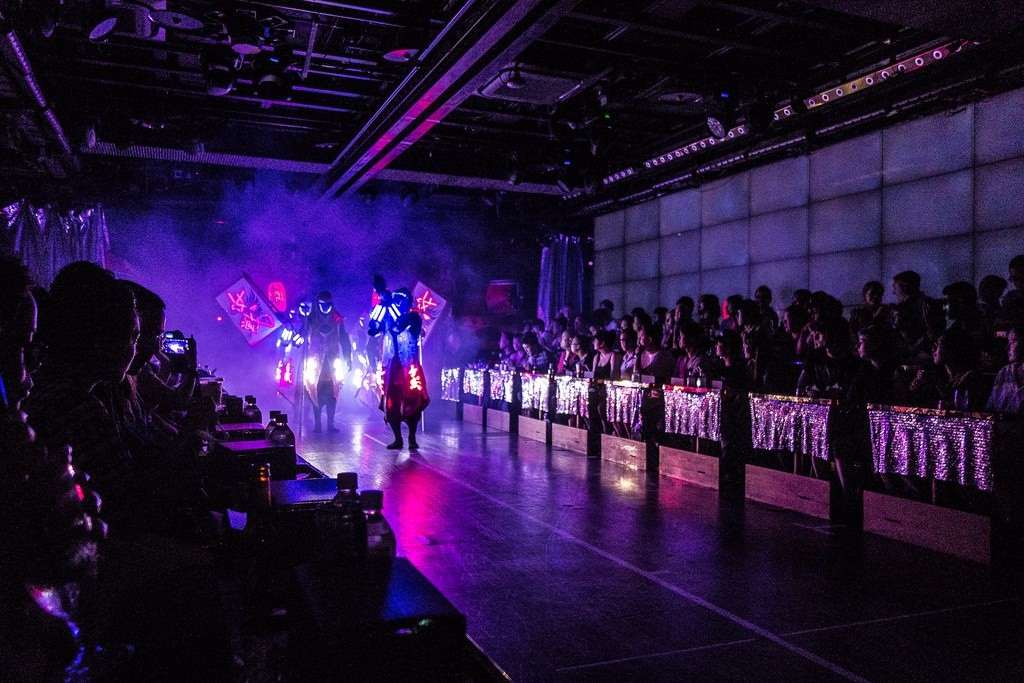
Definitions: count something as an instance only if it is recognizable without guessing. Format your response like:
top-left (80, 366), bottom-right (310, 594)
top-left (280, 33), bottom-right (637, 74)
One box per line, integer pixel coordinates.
top-left (954, 388), bottom-right (968, 410)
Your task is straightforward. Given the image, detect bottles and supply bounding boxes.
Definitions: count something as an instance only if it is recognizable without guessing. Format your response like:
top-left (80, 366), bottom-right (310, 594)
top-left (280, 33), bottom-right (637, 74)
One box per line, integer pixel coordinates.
top-left (198, 364), bottom-right (211, 377)
top-left (264, 411), bottom-right (296, 479)
top-left (243, 395), bottom-right (261, 423)
top-left (686, 370), bottom-right (707, 387)
top-left (499, 360), bottom-right (508, 372)
top-left (332, 472), bottom-right (396, 582)
top-left (547, 363), bottom-right (555, 377)
top-left (631, 369), bottom-right (640, 382)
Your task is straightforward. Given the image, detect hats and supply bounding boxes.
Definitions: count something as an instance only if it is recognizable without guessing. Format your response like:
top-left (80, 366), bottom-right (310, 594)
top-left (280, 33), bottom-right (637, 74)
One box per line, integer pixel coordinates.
top-left (717, 330), bottom-right (741, 349)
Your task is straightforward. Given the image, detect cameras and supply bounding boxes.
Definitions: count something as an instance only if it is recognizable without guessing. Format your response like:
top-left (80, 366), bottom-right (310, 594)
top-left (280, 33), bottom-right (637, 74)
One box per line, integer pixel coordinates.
top-left (161, 331), bottom-right (191, 357)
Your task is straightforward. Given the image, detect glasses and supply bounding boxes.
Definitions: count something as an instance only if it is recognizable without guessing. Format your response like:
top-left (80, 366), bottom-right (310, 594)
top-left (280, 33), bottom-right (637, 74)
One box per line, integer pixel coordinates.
top-left (141, 329), bottom-right (166, 349)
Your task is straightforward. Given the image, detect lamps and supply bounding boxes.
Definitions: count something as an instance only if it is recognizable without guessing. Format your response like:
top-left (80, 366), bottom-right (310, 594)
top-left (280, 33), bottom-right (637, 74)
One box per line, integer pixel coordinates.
top-left (706, 106), bottom-right (735, 139)
top-left (507, 72), bottom-right (525, 88)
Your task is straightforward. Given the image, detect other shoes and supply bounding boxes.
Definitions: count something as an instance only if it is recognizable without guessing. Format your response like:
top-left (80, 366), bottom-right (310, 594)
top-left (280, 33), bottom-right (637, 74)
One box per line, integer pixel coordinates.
top-left (386, 438), bottom-right (402, 449)
top-left (327, 423), bottom-right (339, 432)
top-left (313, 423), bottom-right (322, 433)
top-left (408, 437), bottom-right (419, 450)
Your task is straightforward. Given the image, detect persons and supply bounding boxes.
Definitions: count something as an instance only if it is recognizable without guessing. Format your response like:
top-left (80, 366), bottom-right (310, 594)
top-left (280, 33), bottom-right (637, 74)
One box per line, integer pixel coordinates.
top-left (0, 253), bottom-right (218, 683)
top-left (438, 252), bottom-right (1024, 507)
top-left (274, 286), bottom-right (431, 450)
